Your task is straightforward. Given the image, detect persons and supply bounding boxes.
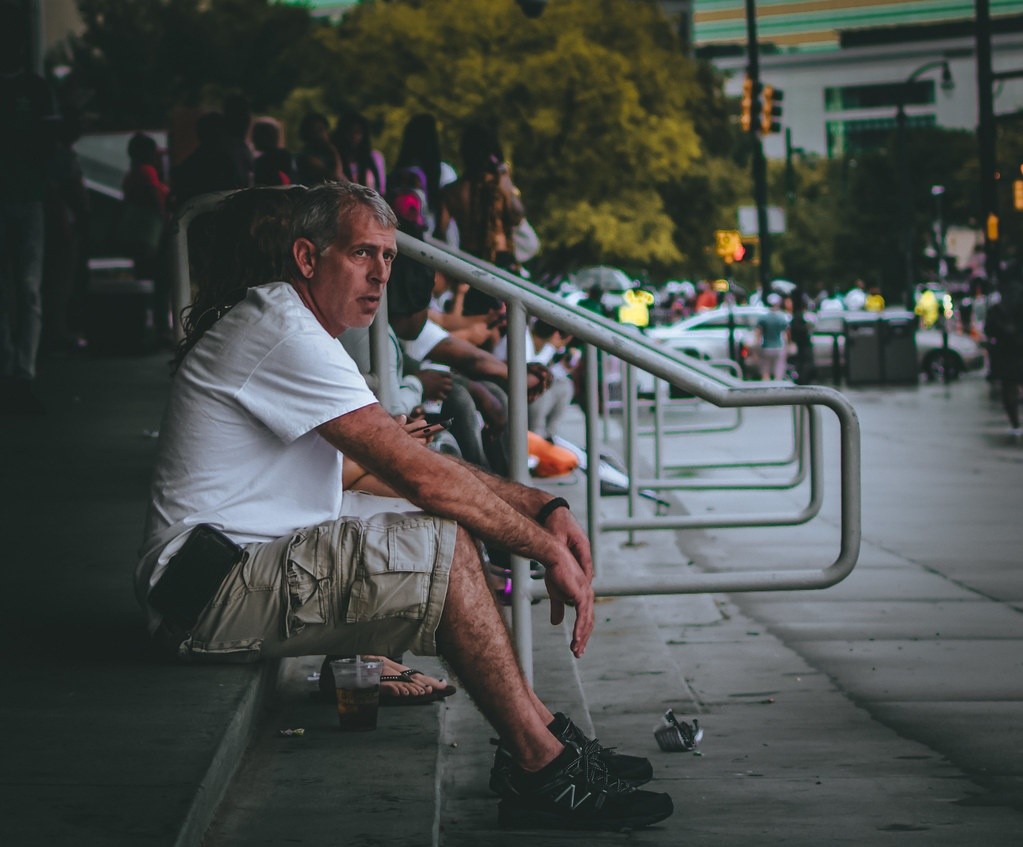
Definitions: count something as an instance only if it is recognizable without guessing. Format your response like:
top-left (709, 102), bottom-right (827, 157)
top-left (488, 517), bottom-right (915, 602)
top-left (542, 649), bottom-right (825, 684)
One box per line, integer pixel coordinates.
top-left (38, 93), bottom-right (1004, 705)
top-left (135, 181), bottom-right (675, 828)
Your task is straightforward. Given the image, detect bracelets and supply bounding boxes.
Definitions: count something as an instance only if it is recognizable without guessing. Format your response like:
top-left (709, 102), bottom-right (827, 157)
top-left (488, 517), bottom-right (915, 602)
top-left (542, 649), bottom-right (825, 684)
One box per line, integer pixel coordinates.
top-left (536, 497), bottom-right (570, 526)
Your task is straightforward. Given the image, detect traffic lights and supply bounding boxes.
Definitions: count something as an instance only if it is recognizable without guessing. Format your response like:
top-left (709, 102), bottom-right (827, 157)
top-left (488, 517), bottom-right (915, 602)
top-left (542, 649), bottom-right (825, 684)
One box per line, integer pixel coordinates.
top-left (730, 237), bottom-right (761, 264)
top-left (761, 82), bottom-right (785, 133)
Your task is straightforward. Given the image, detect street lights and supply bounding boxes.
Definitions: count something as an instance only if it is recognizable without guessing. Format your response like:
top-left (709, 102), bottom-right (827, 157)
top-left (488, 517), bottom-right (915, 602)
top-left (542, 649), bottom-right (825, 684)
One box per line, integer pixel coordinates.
top-left (895, 60), bottom-right (957, 309)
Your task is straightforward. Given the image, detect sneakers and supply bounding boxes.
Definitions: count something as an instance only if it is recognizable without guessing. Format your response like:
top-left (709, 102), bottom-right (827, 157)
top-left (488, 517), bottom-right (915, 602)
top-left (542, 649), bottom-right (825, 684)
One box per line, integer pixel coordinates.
top-left (496, 742), bottom-right (674, 827)
top-left (486, 711), bottom-right (655, 788)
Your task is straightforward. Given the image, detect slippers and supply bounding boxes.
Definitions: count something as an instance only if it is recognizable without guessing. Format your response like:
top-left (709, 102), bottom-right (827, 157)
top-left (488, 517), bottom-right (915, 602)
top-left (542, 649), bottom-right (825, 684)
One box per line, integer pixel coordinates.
top-left (399, 666), bottom-right (456, 698)
top-left (309, 658), bottom-right (438, 706)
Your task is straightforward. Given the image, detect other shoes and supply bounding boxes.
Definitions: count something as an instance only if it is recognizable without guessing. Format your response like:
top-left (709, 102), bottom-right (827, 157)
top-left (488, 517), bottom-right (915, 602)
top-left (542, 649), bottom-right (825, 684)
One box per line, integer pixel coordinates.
top-left (491, 568), bottom-right (545, 608)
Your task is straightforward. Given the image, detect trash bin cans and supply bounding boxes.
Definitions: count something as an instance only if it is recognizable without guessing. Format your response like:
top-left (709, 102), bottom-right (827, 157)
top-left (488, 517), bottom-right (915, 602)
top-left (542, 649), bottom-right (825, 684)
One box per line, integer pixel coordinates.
top-left (881, 316), bottom-right (919, 386)
top-left (841, 317), bottom-right (882, 386)
top-left (81, 256), bottom-right (155, 354)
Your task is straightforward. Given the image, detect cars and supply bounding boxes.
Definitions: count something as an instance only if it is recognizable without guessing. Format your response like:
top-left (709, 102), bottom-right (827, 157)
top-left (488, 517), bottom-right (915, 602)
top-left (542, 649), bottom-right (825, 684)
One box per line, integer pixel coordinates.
top-left (806, 310), bottom-right (985, 384)
top-left (634, 304), bottom-right (845, 401)
